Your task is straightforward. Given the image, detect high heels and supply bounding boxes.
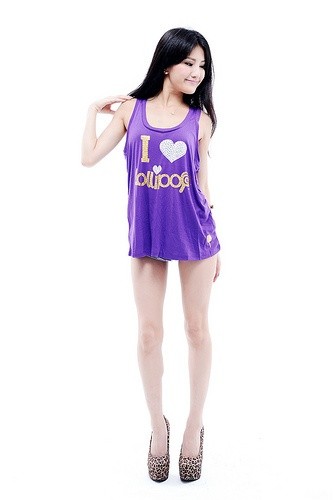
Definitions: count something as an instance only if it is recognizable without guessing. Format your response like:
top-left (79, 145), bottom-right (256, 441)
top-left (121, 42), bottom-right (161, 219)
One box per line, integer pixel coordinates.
top-left (179, 427), bottom-right (203, 481)
top-left (147, 415), bottom-right (171, 482)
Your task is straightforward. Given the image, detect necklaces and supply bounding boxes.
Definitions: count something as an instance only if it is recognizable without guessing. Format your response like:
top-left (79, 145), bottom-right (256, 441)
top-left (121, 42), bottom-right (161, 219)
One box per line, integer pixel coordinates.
top-left (159, 97), bottom-right (183, 116)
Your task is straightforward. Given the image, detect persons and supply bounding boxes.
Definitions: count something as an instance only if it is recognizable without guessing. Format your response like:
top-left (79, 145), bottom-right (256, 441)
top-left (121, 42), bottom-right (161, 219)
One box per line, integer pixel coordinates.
top-left (80, 27), bottom-right (225, 484)
